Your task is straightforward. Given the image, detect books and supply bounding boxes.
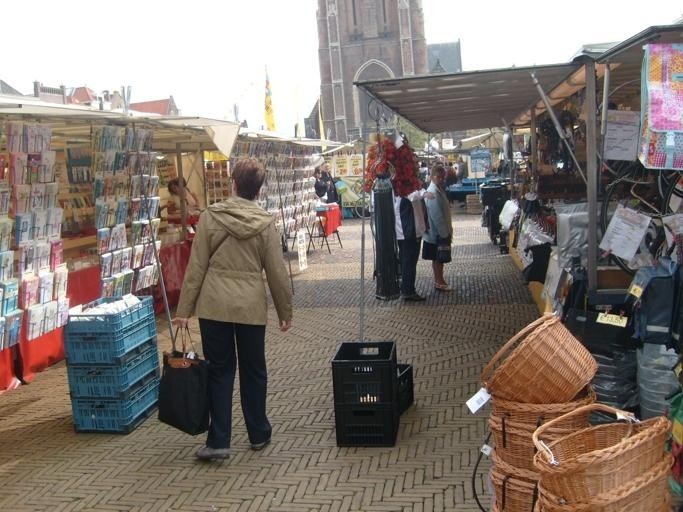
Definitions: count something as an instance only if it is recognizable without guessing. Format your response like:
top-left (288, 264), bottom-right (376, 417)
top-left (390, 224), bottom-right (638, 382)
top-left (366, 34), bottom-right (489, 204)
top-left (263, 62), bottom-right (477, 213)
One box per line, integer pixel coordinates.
top-left (0, 111), bottom-right (165, 349)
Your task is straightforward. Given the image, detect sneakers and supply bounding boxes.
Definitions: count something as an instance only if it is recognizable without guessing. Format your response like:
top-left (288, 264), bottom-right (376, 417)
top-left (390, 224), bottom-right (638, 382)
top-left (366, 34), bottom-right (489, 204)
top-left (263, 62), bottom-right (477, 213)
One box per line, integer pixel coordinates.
top-left (196, 447), bottom-right (229, 459)
top-left (251, 438), bottom-right (271, 450)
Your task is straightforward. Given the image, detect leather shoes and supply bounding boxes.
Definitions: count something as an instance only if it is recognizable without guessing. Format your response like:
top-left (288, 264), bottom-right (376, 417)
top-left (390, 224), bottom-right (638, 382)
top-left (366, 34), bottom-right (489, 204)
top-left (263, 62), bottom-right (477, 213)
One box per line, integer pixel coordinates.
top-left (402, 293), bottom-right (425, 301)
top-left (434, 281), bottom-right (452, 291)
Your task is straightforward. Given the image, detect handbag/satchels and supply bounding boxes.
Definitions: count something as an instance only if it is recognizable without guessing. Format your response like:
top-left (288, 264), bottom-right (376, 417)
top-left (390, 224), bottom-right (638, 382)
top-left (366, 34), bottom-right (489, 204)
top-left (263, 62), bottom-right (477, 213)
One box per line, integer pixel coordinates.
top-left (158, 323), bottom-right (210, 436)
top-left (557, 217), bottom-right (683, 354)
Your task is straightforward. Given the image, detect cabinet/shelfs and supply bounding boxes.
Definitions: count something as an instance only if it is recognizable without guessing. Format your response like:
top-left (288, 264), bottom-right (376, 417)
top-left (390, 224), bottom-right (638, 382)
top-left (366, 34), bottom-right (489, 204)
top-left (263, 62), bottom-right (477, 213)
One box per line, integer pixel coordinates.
top-left (206, 160), bottom-right (231, 205)
top-left (0, 137), bottom-right (169, 275)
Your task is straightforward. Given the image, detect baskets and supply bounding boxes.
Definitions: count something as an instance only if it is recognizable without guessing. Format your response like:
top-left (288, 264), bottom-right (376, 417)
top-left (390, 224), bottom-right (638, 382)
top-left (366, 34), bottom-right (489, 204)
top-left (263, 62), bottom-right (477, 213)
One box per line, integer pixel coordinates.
top-left (331, 341), bottom-right (413, 446)
top-left (479, 313), bottom-right (675, 512)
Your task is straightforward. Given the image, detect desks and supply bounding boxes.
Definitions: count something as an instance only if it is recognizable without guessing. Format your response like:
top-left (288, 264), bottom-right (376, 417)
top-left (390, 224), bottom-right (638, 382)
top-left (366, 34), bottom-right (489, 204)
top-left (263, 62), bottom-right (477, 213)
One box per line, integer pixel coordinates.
top-left (306, 206), bottom-right (343, 255)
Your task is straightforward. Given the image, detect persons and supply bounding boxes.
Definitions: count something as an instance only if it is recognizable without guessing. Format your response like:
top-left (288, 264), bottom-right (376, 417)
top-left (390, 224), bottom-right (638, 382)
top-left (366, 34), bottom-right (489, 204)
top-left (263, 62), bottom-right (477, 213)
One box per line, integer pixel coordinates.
top-left (422, 165), bottom-right (459, 295)
top-left (444, 161), bottom-right (455, 179)
top-left (169, 157), bottom-right (293, 460)
top-left (166, 177), bottom-right (198, 213)
top-left (157, 200), bottom-right (174, 215)
top-left (497, 159), bottom-right (505, 177)
top-left (321, 161), bottom-right (336, 209)
top-left (393, 179), bottom-right (431, 302)
top-left (313, 167), bottom-right (329, 202)
